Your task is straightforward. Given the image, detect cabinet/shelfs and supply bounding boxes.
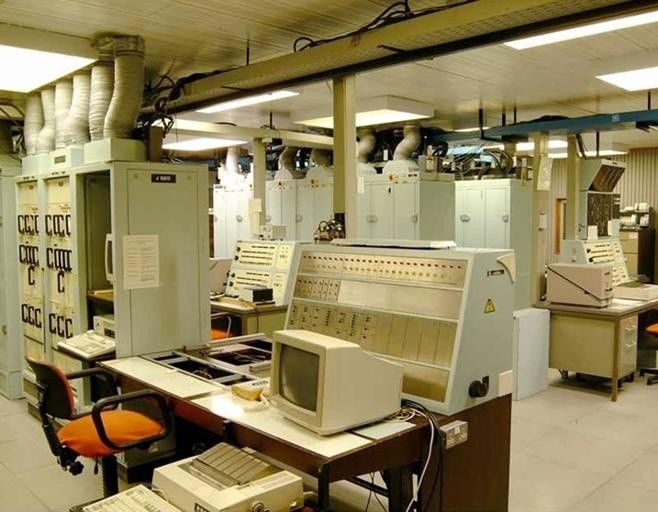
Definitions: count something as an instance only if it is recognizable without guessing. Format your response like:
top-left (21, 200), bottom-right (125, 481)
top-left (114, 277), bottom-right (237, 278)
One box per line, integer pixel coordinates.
top-left (619, 225), bottom-right (655, 283)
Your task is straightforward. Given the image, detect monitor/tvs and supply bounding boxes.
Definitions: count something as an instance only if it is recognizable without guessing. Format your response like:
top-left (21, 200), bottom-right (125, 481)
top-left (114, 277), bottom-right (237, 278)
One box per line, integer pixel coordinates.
top-left (104, 233), bottom-right (113, 284)
top-left (268, 329), bottom-right (404, 437)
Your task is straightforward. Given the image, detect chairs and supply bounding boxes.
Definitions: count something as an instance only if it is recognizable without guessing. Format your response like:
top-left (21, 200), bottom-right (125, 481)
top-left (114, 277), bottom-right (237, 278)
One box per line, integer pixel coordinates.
top-left (23, 353), bottom-right (171, 512)
top-left (639, 323), bottom-right (658, 384)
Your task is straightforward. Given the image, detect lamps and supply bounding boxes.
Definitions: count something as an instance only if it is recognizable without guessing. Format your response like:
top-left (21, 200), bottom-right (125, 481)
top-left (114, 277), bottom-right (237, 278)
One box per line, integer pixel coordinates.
top-left (501, 0), bottom-right (658, 93)
top-left (162, 132), bottom-right (249, 152)
top-left (448, 128), bottom-right (628, 158)
top-left (0, 21), bottom-right (98, 95)
top-left (291, 94), bottom-right (435, 129)
top-left (196, 86), bottom-right (301, 115)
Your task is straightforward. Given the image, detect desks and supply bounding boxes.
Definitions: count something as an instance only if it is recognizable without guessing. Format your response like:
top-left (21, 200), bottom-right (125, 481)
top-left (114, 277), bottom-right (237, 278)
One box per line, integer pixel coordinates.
top-left (95, 334), bottom-right (511, 512)
top-left (531, 284), bottom-right (658, 401)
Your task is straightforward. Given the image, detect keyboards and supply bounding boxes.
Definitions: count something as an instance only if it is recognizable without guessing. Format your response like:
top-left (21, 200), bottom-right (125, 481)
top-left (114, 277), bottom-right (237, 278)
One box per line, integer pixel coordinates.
top-left (82, 484), bottom-right (183, 512)
top-left (56, 331), bottom-right (116, 360)
